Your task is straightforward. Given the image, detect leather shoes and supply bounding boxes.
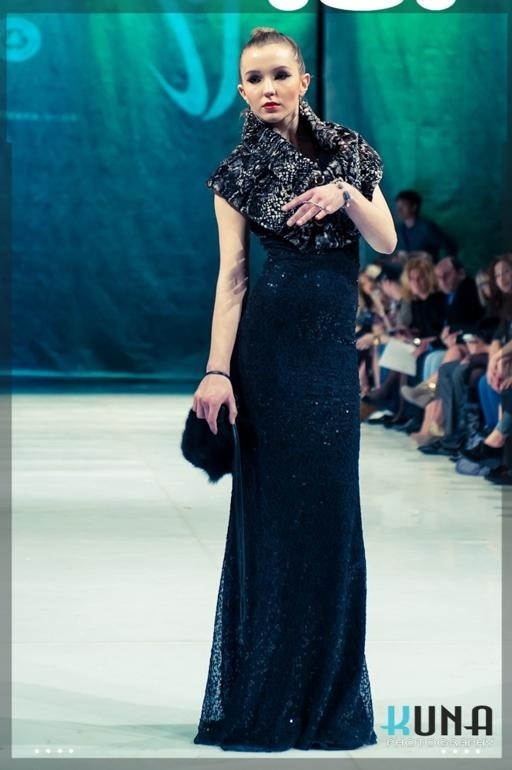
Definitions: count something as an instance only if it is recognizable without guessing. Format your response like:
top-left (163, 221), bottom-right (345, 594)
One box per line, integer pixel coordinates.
top-left (359, 380), bottom-right (509, 486)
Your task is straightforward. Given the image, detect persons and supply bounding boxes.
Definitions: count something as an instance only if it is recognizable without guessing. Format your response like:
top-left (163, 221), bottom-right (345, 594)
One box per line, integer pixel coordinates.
top-left (193, 27), bottom-right (398, 752)
top-left (355, 190), bottom-right (512, 484)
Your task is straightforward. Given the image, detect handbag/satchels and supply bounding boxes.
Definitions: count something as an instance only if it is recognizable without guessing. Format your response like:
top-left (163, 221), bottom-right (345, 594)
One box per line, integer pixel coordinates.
top-left (182, 404), bottom-right (236, 484)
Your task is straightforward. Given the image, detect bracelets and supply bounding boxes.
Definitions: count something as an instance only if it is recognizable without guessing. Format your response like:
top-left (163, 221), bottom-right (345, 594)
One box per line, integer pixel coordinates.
top-left (204, 371), bottom-right (231, 380)
top-left (330, 177), bottom-right (350, 209)
